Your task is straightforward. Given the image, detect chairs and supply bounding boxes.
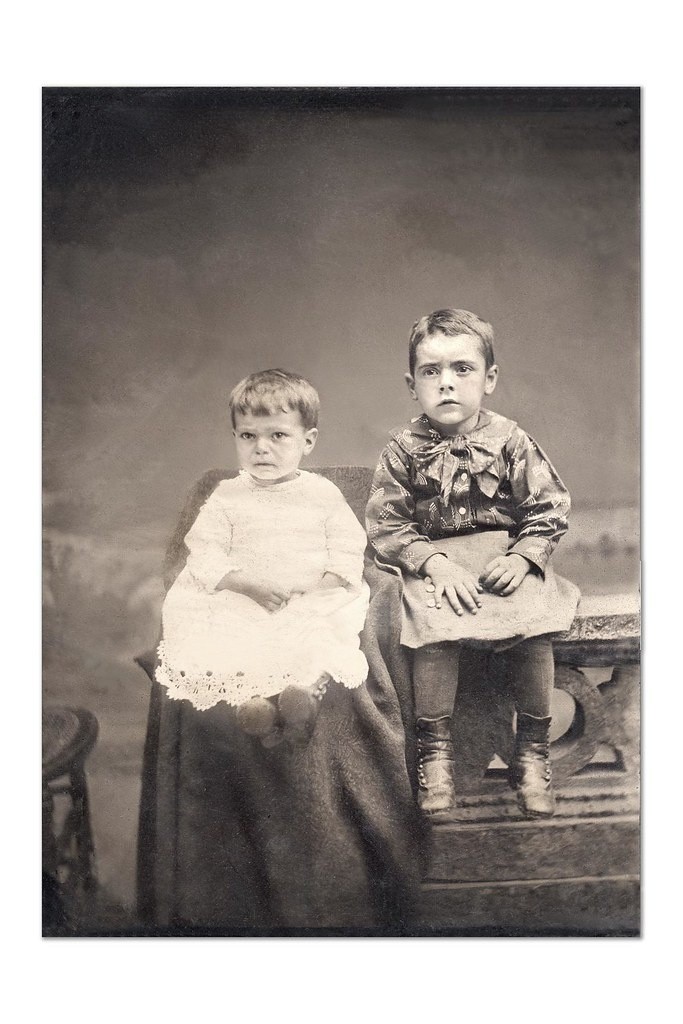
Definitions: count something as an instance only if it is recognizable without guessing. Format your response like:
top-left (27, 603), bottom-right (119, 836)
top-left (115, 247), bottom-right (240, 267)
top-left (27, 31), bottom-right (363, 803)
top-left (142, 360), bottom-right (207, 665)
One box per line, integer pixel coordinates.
top-left (133, 466), bottom-right (373, 920)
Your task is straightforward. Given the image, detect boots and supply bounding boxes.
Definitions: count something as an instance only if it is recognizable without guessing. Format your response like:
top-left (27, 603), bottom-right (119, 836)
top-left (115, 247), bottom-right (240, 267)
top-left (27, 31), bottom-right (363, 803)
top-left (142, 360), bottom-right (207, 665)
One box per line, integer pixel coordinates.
top-left (510, 713), bottom-right (554, 817)
top-left (413, 715), bottom-right (456, 812)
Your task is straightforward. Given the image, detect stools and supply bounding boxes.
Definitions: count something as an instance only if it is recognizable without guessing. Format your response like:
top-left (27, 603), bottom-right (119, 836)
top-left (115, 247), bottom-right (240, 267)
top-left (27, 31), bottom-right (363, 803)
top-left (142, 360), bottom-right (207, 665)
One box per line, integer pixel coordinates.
top-left (42, 701), bottom-right (100, 928)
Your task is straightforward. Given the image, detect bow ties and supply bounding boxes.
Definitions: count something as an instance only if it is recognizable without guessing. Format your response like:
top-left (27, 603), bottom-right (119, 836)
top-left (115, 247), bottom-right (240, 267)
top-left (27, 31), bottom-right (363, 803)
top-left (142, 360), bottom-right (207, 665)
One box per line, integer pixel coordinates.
top-left (414, 439), bottom-right (500, 507)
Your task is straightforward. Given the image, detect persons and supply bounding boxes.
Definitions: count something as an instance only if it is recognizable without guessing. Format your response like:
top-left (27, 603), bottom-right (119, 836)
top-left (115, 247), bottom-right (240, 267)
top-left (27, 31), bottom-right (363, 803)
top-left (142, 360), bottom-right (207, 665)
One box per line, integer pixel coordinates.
top-left (152, 366), bottom-right (373, 757)
top-left (364, 307), bottom-right (582, 823)
